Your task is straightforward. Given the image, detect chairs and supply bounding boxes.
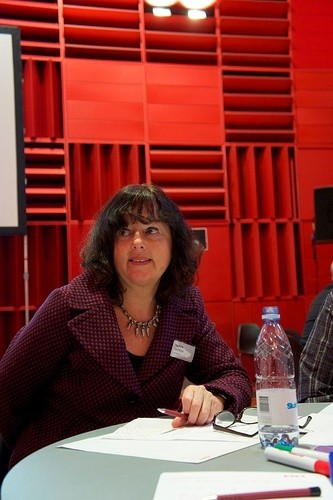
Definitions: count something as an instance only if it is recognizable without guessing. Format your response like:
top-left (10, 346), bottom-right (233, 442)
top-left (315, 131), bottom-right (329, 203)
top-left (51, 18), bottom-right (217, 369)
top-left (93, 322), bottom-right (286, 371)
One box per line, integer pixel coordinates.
top-left (237, 322), bottom-right (303, 371)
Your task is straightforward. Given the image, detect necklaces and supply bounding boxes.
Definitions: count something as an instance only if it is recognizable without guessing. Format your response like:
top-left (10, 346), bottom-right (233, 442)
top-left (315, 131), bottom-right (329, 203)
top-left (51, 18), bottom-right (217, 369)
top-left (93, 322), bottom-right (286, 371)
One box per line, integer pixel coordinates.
top-left (122, 303), bottom-right (161, 337)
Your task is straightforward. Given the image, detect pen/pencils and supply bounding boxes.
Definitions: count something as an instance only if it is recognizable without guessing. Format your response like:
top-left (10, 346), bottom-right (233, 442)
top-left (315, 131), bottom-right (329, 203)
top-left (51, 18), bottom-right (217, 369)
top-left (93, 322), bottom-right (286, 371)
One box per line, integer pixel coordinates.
top-left (158, 407), bottom-right (188, 420)
top-left (216, 486), bottom-right (321, 500)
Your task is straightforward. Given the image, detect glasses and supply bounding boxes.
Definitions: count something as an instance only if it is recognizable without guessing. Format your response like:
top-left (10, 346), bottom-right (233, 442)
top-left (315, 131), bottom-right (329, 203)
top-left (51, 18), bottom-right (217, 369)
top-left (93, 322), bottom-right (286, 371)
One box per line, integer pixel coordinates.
top-left (213, 405), bottom-right (312, 438)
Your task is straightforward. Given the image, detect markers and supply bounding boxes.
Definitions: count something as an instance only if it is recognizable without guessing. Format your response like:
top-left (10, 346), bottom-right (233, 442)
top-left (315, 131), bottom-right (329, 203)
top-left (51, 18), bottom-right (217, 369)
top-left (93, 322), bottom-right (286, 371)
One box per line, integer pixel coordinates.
top-left (264, 444), bottom-right (330, 477)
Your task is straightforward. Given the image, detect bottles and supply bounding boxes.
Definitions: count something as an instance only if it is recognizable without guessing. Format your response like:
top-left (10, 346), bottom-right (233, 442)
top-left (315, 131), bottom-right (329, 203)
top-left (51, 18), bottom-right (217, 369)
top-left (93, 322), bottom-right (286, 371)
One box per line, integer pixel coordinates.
top-left (253, 305), bottom-right (300, 450)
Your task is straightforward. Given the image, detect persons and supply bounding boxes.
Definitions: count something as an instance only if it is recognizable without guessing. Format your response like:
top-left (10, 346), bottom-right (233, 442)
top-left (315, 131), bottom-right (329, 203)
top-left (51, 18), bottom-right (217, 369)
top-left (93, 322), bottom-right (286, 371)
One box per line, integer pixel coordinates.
top-left (0, 184), bottom-right (253, 486)
top-left (297, 260), bottom-right (333, 402)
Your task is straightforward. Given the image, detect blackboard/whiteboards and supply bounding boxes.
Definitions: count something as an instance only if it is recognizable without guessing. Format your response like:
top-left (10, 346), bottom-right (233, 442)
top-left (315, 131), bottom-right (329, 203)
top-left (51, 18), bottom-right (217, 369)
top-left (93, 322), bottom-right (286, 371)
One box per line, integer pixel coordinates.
top-left (0, 24), bottom-right (27, 235)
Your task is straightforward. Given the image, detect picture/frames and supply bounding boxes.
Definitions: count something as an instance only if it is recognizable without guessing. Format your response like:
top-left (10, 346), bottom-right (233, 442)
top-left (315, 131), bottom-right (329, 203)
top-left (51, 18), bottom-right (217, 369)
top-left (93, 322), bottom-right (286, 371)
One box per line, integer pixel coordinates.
top-left (0, 25), bottom-right (26, 232)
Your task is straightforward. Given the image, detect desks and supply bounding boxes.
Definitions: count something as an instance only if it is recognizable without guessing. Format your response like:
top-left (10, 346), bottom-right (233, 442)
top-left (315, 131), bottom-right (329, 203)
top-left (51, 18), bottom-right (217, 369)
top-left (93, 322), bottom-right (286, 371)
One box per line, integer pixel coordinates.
top-left (0, 401), bottom-right (333, 500)
